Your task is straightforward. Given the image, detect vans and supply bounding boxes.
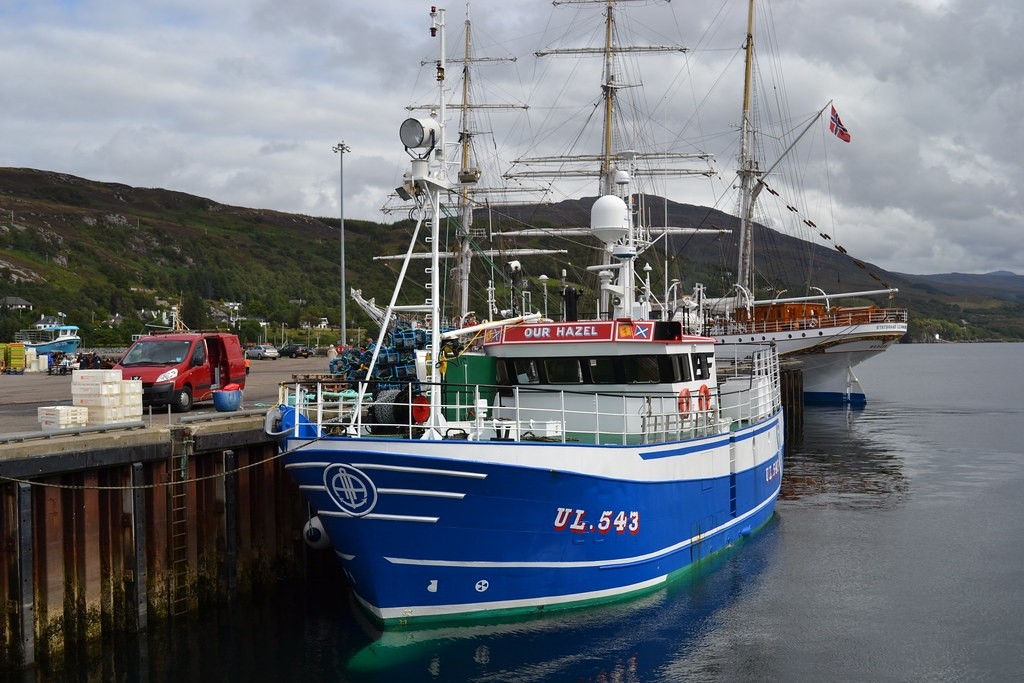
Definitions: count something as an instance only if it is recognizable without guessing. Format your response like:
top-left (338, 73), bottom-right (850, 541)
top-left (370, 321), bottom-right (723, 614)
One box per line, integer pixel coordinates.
top-left (113, 332), bottom-right (246, 413)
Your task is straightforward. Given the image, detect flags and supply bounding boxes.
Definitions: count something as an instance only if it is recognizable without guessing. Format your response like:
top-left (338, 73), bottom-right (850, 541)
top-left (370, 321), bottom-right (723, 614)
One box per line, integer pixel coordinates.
top-left (830, 105), bottom-right (851, 143)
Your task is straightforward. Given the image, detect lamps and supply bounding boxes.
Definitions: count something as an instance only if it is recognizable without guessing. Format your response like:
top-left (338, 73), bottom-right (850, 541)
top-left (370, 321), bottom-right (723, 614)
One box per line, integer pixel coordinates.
top-left (503, 260), bottom-right (522, 274)
top-left (398, 116), bottom-right (440, 159)
top-left (394, 182), bottom-right (417, 201)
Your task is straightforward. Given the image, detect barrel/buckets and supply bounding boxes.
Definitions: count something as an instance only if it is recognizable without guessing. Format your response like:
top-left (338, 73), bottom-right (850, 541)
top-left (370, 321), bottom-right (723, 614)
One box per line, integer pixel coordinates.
top-left (212, 388), bottom-right (242, 411)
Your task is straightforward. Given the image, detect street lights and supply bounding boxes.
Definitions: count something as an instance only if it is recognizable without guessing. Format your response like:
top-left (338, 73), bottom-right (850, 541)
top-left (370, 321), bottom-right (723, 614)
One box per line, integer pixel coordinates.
top-left (332, 141), bottom-right (352, 346)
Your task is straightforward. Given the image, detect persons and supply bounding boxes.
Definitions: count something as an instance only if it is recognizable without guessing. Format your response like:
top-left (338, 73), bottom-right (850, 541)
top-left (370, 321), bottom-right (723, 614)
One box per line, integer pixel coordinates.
top-left (327, 344), bottom-right (338, 361)
top-left (366, 338), bottom-right (373, 349)
top-left (50, 352), bottom-right (101, 369)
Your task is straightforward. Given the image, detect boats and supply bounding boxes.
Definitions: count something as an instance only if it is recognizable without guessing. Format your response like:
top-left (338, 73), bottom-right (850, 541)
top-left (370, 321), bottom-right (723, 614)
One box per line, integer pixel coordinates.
top-left (23, 326), bottom-right (81, 354)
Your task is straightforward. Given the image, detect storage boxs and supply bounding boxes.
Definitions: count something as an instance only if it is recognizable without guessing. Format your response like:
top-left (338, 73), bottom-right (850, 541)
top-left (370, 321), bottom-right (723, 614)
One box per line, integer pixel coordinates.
top-left (24, 347), bottom-right (143, 433)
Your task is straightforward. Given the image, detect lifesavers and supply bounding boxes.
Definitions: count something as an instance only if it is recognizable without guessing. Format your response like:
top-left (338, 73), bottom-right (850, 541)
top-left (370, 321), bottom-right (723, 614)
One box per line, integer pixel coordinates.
top-left (698, 384), bottom-right (711, 412)
top-left (678, 388), bottom-right (692, 419)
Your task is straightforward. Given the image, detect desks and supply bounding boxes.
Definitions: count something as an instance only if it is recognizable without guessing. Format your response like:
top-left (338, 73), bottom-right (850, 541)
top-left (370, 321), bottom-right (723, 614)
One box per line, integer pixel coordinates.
top-left (468, 420), bottom-right (566, 438)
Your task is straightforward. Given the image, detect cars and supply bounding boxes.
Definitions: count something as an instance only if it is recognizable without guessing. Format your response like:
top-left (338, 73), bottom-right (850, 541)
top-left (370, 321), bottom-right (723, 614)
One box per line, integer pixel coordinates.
top-left (277, 344), bottom-right (309, 358)
top-left (245, 345), bottom-right (279, 360)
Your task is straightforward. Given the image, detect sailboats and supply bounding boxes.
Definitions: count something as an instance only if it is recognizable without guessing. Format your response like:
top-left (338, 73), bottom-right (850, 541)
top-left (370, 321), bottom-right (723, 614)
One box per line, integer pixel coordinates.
top-left (276, 1), bottom-right (908, 630)
